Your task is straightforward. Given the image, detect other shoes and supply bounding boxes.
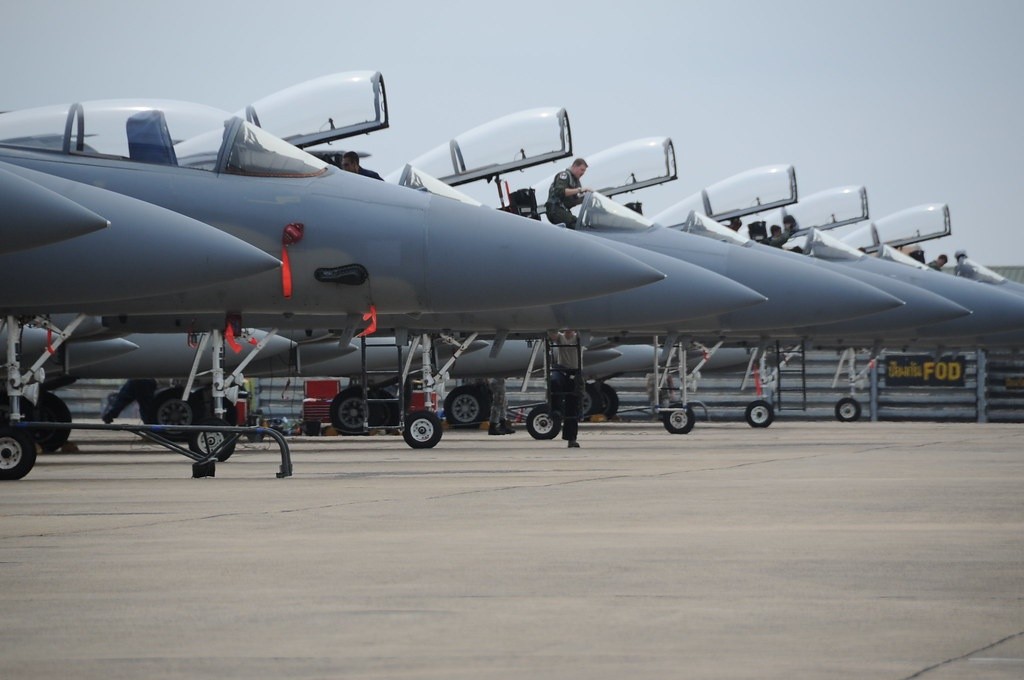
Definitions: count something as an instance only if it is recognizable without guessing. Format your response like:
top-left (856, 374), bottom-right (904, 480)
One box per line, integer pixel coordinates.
top-left (568, 443), bottom-right (579, 448)
top-left (552, 410), bottom-right (562, 418)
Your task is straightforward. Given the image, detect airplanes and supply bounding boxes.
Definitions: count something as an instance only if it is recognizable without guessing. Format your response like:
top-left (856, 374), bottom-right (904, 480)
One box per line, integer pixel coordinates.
top-left (0, 70), bottom-right (1024, 483)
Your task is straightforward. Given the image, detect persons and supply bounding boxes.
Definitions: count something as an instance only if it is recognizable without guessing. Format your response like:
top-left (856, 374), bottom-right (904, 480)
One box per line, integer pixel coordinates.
top-left (953, 250), bottom-right (969, 274)
top-left (488, 377), bottom-right (516, 435)
top-left (548, 329), bottom-right (585, 448)
top-left (766, 216), bottom-right (803, 253)
top-left (545, 158), bottom-right (593, 229)
top-left (342, 151), bottom-right (384, 181)
top-left (926, 254), bottom-right (948, 271)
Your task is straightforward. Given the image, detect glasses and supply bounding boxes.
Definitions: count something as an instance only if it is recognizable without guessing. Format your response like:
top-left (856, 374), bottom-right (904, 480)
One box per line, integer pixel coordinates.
top-left (342, 162), bottom-right (350, 167)
